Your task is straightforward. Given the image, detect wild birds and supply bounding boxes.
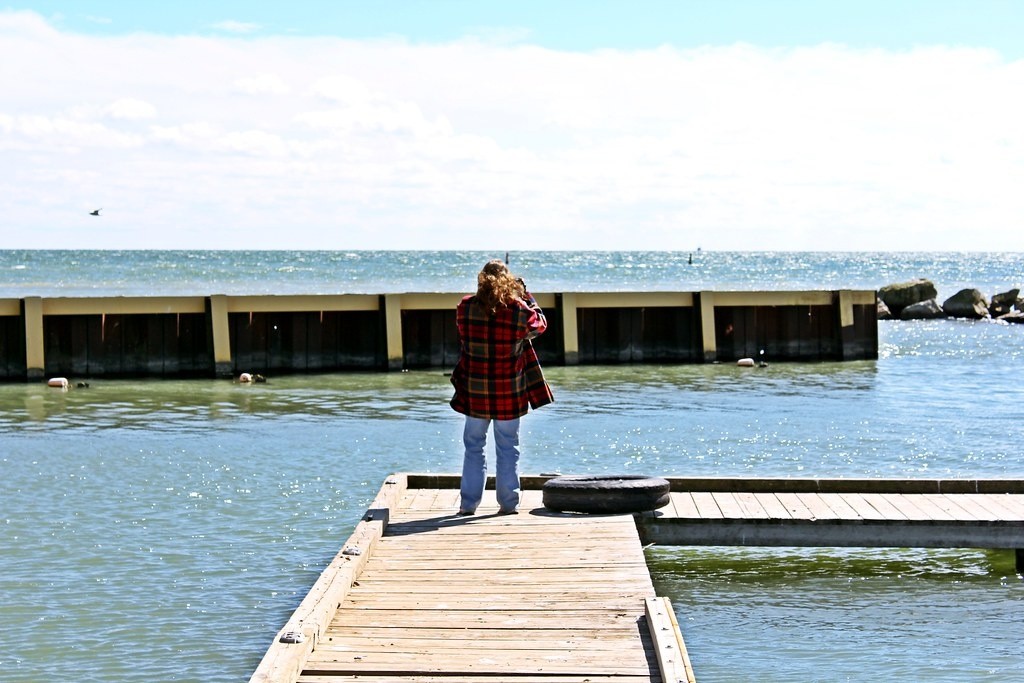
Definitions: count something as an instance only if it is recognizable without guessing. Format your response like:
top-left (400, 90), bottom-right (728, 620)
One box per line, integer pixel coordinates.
top-left (88, 208), bottom-right (103, 217)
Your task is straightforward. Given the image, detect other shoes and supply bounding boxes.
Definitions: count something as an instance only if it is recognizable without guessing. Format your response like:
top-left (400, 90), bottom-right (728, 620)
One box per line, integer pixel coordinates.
top-left (500, 508), bottom-right (516, 514)
top-left (459, 506), bottom-right (474, 515)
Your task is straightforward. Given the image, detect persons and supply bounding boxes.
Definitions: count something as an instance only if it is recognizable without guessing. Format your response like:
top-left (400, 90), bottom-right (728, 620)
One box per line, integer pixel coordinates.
top-left (448, 259), bottom-right (554, 515)
